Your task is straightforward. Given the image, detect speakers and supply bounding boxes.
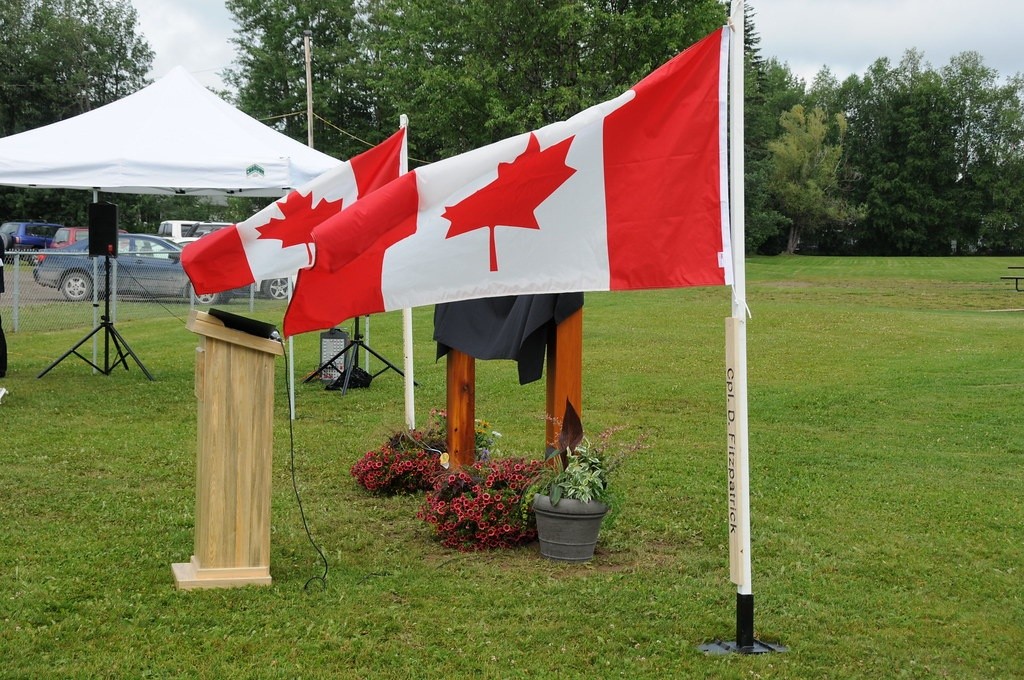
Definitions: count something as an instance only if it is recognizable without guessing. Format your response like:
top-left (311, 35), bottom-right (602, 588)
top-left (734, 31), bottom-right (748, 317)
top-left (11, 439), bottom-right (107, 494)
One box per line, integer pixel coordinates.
top-left (88, 201), bottom-right (119, 257)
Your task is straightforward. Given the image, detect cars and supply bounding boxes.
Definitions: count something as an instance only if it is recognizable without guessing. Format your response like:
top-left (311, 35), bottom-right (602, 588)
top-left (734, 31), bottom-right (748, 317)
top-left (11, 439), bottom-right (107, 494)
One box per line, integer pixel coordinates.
top-left (33, 232), bottom-right (251, 307)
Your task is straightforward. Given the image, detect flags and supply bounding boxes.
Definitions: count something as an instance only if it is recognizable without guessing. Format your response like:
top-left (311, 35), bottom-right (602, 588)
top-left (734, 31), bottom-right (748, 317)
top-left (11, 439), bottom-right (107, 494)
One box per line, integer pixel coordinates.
top-left (282, 23), bottom-right (732, 341)
top-left (180, 118), bottom-right (403, 298)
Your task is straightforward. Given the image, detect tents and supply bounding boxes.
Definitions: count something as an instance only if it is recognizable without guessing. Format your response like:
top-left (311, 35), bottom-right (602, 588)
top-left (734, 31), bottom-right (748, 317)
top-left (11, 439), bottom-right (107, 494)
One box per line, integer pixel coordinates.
top-left (0, 65), bottom-right (371, 374)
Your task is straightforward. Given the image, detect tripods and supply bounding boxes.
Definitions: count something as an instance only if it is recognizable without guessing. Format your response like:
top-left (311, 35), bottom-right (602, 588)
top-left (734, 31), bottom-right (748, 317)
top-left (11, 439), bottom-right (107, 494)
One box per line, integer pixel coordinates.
top-left (303, 315), bottom-right (417, 399)
top-left (37, 255), bottom-right (154, 381)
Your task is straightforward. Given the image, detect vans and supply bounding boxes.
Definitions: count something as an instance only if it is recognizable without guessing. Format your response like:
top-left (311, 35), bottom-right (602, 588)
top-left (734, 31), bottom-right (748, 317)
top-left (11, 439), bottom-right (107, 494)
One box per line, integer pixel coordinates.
top-left (51, 227), bottom-right (130, 249)
top-left (140, 220), bottom-right (292, 299)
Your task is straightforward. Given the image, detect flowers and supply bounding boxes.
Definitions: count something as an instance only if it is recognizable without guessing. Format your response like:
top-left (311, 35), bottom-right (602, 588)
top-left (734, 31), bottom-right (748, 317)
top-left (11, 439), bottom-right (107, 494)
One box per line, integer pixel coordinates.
top-left (417, 457), bottom-right (549, 554)
top-left (350, 413), bottom-right (503, 494)
top-left (532, 412), bottom-right (654, 506)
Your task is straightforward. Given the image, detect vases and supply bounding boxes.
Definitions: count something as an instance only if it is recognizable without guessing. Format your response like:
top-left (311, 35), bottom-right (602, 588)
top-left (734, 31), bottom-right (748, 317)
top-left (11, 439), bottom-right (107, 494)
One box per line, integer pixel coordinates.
top-left (531, 494), bottom-right (610, 563)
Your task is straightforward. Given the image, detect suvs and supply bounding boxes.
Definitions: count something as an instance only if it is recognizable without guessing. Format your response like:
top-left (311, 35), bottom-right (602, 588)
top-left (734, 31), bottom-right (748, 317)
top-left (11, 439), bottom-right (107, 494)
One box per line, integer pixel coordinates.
top-left (1, 219), bottom-right (67, 267)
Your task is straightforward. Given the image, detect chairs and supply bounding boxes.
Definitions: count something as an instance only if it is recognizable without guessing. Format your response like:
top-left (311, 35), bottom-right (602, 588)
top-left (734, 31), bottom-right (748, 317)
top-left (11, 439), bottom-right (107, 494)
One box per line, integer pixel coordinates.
top-left (139, 241), bottom-right (156, 257)
